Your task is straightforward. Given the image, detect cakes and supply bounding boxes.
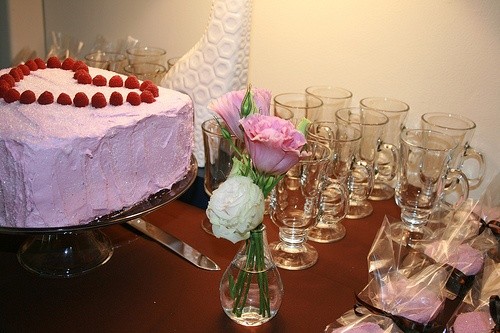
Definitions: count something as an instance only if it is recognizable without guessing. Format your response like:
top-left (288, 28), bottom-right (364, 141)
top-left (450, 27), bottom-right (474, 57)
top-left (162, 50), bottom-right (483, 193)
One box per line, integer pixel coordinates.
top-left (0, 56), bottom-right (194, 226)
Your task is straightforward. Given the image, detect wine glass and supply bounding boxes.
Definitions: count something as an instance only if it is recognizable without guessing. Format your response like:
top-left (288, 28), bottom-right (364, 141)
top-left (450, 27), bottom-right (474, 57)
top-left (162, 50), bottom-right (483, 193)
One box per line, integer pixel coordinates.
top-left (406, 113), bottom-right (487, 223)
top-left (382, 130), bottom-right (470, 250)
top-left (200, 84), bottom-right (412, 271)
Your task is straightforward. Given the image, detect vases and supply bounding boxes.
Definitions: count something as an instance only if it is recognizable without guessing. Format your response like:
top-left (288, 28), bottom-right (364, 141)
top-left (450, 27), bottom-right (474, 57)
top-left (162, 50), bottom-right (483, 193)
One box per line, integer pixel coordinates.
top-left (219, 225), bottom-right (284, 326)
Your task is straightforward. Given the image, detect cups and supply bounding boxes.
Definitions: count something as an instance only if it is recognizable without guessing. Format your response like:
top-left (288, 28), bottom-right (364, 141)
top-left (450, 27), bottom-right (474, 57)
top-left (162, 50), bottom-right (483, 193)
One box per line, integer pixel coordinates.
top-left (83, 46), bottom-right (181, 87)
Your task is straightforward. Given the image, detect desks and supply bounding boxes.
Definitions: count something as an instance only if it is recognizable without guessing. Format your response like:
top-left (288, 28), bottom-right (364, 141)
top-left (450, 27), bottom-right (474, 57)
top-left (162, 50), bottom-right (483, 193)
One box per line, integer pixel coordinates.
top-left (0, 156), bottom-right (438, 333)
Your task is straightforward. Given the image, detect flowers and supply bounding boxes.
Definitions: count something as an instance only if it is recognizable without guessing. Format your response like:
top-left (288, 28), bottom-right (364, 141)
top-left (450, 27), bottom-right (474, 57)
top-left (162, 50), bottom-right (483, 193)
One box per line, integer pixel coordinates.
top-left (206, 89), bottom-right (311, 318)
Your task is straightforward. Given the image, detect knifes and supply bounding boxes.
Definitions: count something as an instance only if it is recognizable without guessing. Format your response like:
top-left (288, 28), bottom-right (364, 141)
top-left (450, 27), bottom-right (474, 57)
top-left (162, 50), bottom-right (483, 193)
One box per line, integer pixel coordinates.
top-left (126, 218), bottom-right (221, 270)
